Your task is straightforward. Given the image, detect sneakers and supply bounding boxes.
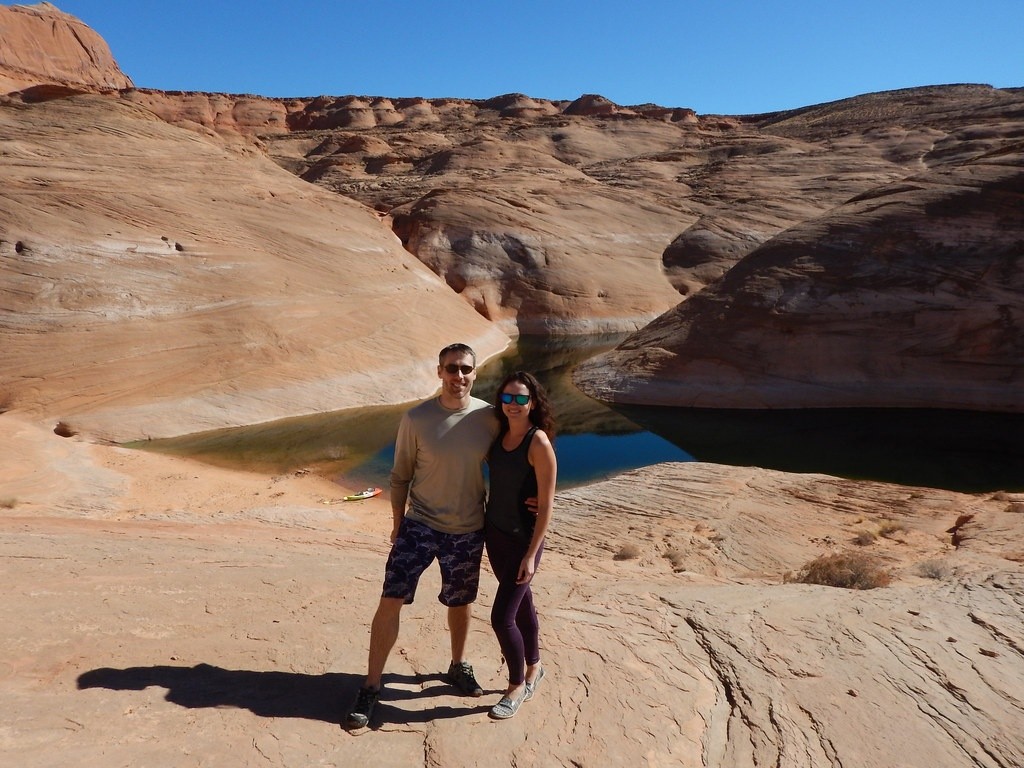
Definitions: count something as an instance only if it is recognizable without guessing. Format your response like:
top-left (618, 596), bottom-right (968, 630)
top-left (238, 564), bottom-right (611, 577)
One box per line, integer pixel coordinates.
top-left (347, 678), bottom-right (384, 728)
top-left (447, 659), bottom-right (483, 696)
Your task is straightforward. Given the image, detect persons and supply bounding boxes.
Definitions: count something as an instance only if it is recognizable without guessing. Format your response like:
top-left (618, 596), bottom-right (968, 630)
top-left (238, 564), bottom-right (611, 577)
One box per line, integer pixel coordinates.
top-left (483, 371), bottom-right (557, 718)
top-left (345, 344), bottom-right (539, 727)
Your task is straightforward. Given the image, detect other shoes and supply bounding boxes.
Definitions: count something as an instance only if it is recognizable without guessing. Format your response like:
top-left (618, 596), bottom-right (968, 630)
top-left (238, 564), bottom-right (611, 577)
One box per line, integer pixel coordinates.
top-left (523, 667), bottom-right (546, 702)
top-left (490, 683), bottom-right (528, 718)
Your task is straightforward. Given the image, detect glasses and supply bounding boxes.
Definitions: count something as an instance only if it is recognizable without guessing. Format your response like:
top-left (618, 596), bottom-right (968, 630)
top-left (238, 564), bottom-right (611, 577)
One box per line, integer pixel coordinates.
top-left (498, 393), bottom-right (533, 406)
top-left (441, 363), bottom-right (474, 375)
